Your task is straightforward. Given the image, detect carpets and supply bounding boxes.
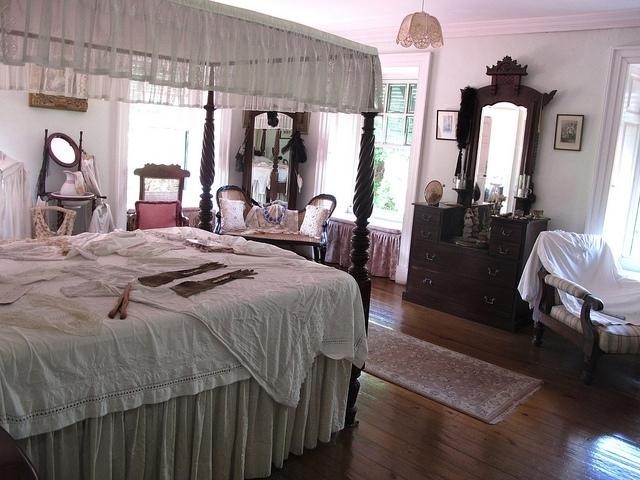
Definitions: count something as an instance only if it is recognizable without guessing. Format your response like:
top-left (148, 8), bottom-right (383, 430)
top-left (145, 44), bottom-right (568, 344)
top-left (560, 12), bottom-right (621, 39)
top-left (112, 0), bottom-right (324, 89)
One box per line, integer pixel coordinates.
top-left (361, 321), bottom-right (545, 427)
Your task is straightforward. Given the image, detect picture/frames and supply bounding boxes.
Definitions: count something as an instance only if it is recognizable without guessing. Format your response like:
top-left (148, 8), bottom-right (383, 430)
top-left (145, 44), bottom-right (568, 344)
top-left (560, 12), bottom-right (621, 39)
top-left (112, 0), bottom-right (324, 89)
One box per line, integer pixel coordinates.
top-left (435, 109), bottom-right (460, 142)
top-left (28, 64), bottom-right (90, 114)
top-left (553, 112), bottom-right (585, 152)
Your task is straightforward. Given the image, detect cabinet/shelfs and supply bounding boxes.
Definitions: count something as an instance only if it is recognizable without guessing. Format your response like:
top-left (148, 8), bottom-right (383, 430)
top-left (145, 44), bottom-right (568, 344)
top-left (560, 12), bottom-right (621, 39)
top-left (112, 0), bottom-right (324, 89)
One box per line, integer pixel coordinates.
top-left (401, 201), bottom-right (551, 334)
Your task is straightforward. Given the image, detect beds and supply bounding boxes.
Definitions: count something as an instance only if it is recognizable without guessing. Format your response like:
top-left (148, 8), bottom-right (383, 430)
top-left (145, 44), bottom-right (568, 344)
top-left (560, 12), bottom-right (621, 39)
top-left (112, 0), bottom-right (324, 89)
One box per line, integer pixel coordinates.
top-left (251, 155), bottom-right (289, 208)
top-left (0, 0), bottom-right (386, 479)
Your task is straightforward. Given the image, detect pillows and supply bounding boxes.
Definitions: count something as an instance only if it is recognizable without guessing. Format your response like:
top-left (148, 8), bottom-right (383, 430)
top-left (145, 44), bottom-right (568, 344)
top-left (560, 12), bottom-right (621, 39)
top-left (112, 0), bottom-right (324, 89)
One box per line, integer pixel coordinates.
top-left (218, 197), bottom-right (247, 233)
top-left (298, 204), bottom-right (329, 241)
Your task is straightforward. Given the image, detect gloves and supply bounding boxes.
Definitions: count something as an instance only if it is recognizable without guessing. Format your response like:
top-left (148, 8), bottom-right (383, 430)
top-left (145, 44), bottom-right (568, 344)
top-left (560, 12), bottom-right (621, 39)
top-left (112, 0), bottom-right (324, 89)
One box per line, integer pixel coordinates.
top-left (169, 269), bottom-right (258, 298)
top-left (138, 261), bottom-right (227, 287)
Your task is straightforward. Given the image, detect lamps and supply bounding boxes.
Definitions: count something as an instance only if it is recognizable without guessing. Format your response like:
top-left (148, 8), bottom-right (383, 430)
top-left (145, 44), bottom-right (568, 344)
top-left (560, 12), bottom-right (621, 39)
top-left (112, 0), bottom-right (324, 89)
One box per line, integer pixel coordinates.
top-left (396, 0), bottom-right (444, 50)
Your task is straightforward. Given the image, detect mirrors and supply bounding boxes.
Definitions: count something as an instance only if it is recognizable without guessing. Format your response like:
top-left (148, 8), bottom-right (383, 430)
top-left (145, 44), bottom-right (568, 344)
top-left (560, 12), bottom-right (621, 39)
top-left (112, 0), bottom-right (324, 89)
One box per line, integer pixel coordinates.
top-left (242, 111), bottom-right (298, 210)
top-left (37, 128), bottom-right (84, 195)
top-left (450, 53), bottom-right (558, 218)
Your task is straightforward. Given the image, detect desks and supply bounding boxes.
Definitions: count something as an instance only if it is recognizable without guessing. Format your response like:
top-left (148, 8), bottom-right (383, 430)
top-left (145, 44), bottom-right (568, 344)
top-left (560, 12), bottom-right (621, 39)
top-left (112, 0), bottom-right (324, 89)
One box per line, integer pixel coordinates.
top-left (45, 188), bottom-right (96, 232)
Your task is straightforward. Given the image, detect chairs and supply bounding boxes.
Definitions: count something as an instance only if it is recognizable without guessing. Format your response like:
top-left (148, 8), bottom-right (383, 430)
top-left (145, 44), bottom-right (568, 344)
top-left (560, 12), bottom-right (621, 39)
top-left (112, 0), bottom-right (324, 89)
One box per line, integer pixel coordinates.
top-left (127, 162), bottom-right (191, 231)
top-left (518, 230), bottom-right (639, 386)
top-left (214, 184), bottom-right (337, 265)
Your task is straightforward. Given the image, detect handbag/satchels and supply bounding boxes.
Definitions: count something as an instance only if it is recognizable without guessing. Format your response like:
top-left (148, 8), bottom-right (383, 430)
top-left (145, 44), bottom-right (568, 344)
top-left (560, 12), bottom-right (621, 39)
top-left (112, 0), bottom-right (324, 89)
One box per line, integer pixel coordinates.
top-left (282, 130), bottom-right (307, 162)
top-left (235, 129), bottom-right (250, 172)
